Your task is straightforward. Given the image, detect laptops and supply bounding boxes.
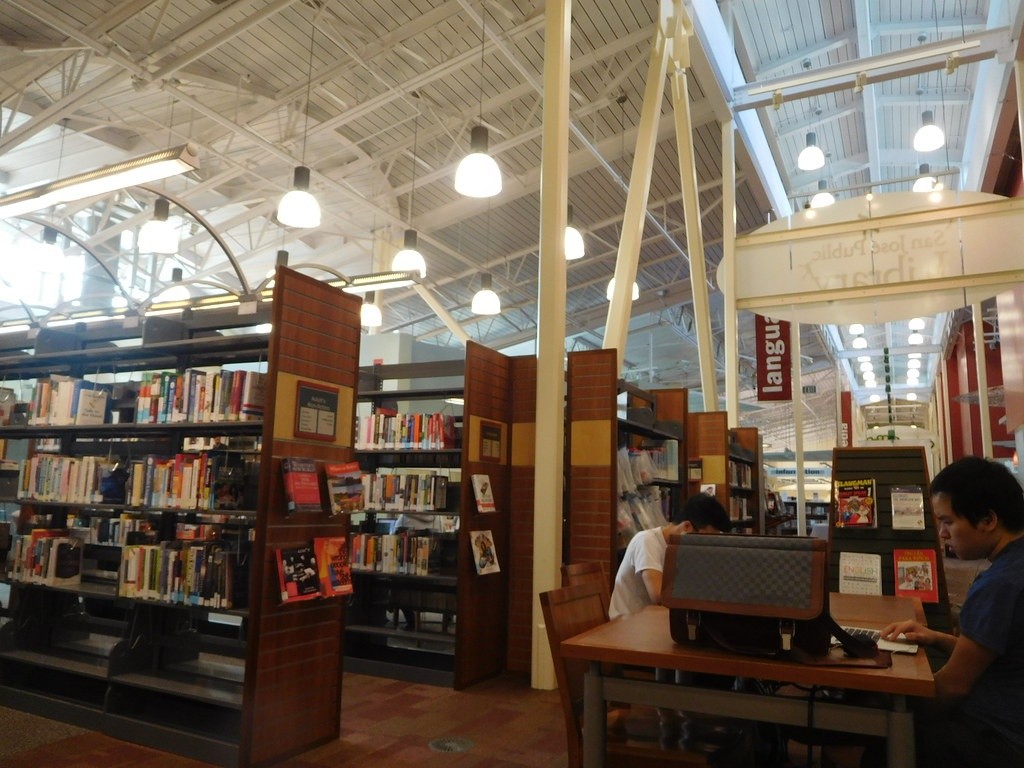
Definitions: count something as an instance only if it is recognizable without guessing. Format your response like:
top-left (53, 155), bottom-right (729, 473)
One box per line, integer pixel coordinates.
top-left (831, 626), bottom-right (919, 655)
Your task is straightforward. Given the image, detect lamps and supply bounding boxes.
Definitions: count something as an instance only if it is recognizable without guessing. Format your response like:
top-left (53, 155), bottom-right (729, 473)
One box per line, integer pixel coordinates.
top-left (0, 0), bottom-right (640, 327)
top-left (797, 35), bottom-right (945, 208)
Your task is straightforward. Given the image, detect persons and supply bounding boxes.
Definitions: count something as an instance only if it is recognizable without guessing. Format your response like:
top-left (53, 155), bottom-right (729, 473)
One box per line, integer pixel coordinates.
top-left (606, 492), bottom-right (730, 750)
top-left (877, 454), bottom-right (1024, 768)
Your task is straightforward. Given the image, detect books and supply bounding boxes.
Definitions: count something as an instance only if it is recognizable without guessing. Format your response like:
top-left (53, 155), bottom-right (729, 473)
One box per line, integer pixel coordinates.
top-left (0, 368), bottom-right (454, 611)
top-left (891, 487), bottom-right (925, 530)
top-left (470, 530), bottom-right (502, 576)
top-left (840, 552), bottom-right (882, 595)
top-left (895, 549), bottom-right (939, 604)
top-left (471, 474), bottom-right (495, 513)
top-left (726, 461), bottom-right (828, 538)
top-left (834, 478), bottom-right (878, 528)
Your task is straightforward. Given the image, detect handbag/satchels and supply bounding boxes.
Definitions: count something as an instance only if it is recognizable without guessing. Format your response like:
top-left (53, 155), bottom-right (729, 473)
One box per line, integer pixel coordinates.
top-left (660, 532), bottom-right (834, 657)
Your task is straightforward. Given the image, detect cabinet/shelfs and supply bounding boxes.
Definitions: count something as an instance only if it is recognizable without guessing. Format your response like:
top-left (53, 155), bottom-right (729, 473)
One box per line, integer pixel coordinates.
top-left (780, 502), bottom-right (830, 534)
top-left (0, 265), bottom-right (363, 768)
top-left (566, 349), bottom-right (688, 589)
top-left (341, 340), bottom-right (512, 690)
top-left (688, 411), bottom-right (761, 535)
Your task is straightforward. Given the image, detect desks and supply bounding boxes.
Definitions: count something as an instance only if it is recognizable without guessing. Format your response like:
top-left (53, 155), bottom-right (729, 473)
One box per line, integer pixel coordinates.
top-left (560, 591), bottom-right (934, 768)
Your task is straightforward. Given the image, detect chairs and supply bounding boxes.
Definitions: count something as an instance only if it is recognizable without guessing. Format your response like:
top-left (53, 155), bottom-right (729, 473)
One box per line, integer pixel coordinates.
top-left (540, 581), bottom-right (708, 768)
top-left (560, 559), bottom-right (610, 602)
